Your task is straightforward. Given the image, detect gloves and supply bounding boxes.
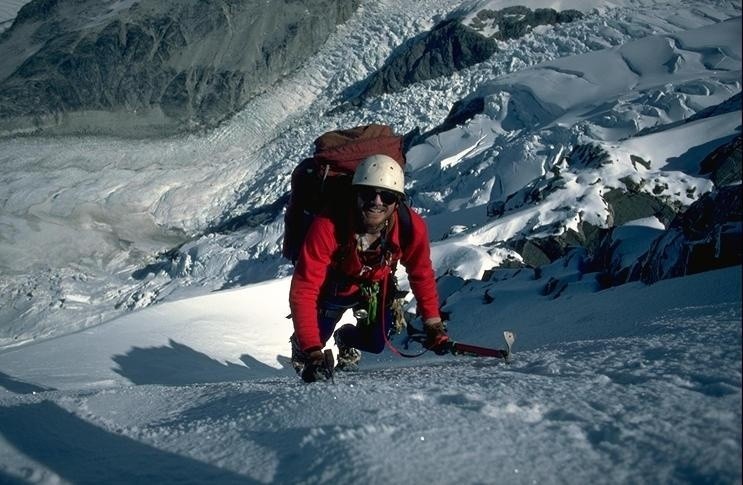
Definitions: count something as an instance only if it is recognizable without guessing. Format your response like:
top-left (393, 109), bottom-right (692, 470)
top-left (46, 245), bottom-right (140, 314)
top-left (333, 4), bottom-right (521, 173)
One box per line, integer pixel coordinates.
top-left (302, 349), bottom-right (330, 381)
top-left (422, 322), bottom-right (450, 355)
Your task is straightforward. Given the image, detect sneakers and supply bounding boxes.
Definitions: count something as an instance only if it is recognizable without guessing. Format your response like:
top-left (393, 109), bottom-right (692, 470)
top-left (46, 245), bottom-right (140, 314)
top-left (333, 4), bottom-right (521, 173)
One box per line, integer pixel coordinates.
top-left (290, 332), bottom-right (304, 376)
top-left (334, 324), bottom-right (360, 373)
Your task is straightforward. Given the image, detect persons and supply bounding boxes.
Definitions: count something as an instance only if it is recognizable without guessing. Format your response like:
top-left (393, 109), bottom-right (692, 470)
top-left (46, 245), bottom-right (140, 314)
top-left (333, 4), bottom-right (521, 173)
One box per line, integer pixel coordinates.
top-left (285, 154), bottom-right (448, 384)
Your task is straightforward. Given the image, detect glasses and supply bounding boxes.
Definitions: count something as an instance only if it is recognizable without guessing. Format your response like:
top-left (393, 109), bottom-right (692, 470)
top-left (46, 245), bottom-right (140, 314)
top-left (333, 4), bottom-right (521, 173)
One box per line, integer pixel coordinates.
top-left (360, 187), bottom-right (399, 205)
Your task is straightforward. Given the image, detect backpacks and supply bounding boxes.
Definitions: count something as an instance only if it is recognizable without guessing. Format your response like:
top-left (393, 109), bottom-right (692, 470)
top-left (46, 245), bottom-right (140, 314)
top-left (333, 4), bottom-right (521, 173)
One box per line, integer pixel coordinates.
top-left (282, 126), bottom-right (413, 260)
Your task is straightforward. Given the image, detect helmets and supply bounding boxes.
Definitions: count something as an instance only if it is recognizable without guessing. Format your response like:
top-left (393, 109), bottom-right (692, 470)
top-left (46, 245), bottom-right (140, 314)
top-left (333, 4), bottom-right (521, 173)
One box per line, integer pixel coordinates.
top-left (352, 155), bottom-right (406, 196)
